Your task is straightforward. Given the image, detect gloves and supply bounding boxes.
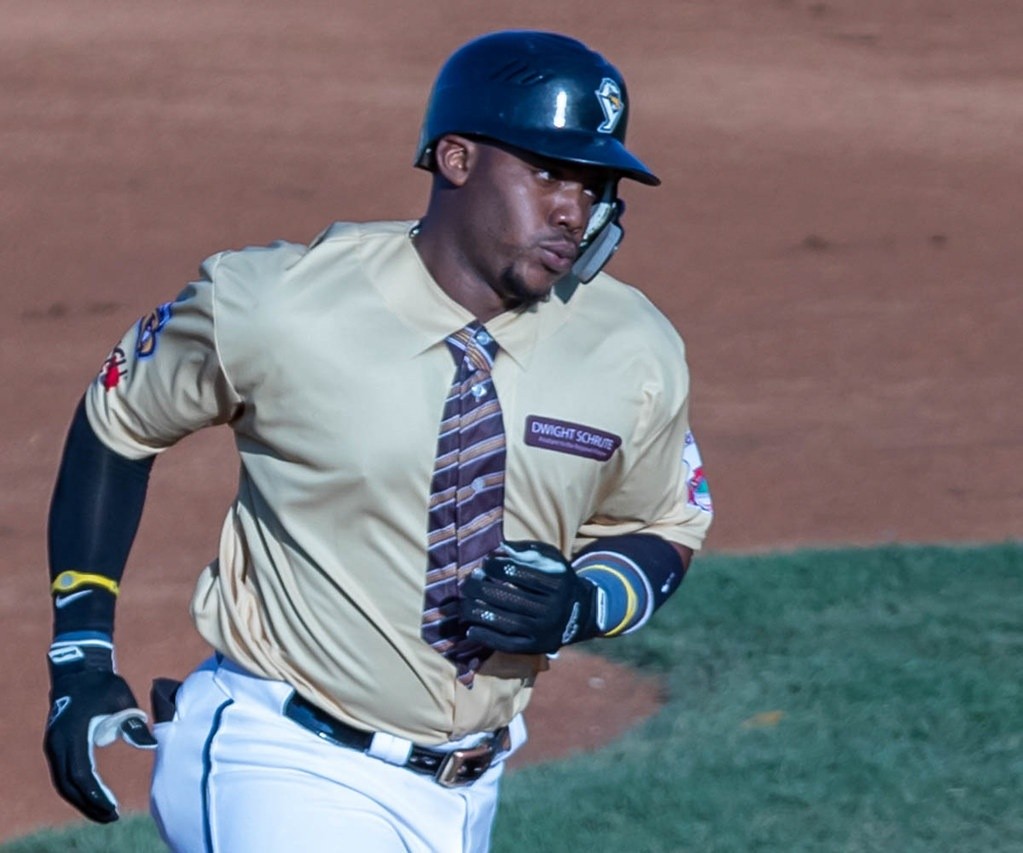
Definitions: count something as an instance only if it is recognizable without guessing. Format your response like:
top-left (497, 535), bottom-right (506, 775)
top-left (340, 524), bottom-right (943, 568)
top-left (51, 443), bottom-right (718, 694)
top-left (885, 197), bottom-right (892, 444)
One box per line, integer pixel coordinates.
top-left (462, 541), bottom-right (607, 652)
top-left (44, 631), bottom-right (158, 824)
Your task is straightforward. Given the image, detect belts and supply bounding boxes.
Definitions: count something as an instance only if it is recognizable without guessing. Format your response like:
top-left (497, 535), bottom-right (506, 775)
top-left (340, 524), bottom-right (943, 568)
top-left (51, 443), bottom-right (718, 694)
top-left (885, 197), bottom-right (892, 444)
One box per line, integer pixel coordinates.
top-left (282, 693), bottom-right (514, 788)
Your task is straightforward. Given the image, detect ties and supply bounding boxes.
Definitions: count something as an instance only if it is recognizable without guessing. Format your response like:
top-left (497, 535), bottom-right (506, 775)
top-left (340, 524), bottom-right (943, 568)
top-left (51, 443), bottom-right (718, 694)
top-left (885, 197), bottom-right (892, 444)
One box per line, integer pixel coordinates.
top-left (420, 328), bottom-right (506, 672)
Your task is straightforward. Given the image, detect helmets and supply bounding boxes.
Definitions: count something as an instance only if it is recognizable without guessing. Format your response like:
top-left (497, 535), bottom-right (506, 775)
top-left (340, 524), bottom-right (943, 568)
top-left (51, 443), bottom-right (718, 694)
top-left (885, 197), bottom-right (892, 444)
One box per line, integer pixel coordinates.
top-left (413, 29), bottom-right (665, 187)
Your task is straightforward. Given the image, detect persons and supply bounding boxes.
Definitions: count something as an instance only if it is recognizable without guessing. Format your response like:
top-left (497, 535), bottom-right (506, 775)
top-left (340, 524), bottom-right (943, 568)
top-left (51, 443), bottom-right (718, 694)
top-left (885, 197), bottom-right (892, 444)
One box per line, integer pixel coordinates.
top-left (41, 30), bottom-right (712, 853)
